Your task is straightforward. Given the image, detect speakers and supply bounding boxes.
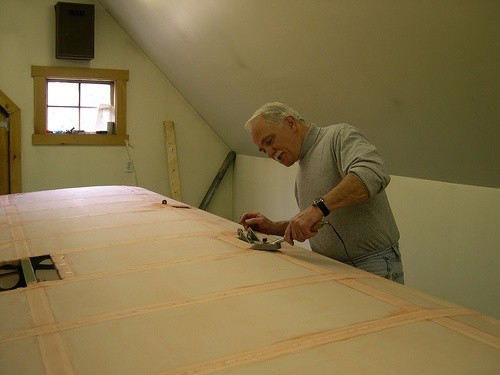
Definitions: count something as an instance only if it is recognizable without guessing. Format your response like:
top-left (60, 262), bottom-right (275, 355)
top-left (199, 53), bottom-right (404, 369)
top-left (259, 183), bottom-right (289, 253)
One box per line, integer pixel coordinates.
top-left (54, 2), bottom-right (94, 59)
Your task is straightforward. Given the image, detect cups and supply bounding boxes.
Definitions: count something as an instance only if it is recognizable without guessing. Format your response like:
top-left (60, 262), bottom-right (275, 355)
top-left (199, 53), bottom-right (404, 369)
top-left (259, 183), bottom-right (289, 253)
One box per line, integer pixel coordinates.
top-left (106, 122), bottom-right (114, 134)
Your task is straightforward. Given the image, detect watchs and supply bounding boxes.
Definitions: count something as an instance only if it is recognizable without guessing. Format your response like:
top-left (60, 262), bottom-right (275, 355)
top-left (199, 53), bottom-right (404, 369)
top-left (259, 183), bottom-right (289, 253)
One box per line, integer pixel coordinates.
top-left (312, 196), bottom-right (330, 216)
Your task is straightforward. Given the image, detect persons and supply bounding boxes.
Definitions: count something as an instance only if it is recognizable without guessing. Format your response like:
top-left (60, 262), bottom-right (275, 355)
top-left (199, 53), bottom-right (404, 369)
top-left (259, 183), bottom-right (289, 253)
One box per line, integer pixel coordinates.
top-left (239, 101), bottom-right (405, 284)
